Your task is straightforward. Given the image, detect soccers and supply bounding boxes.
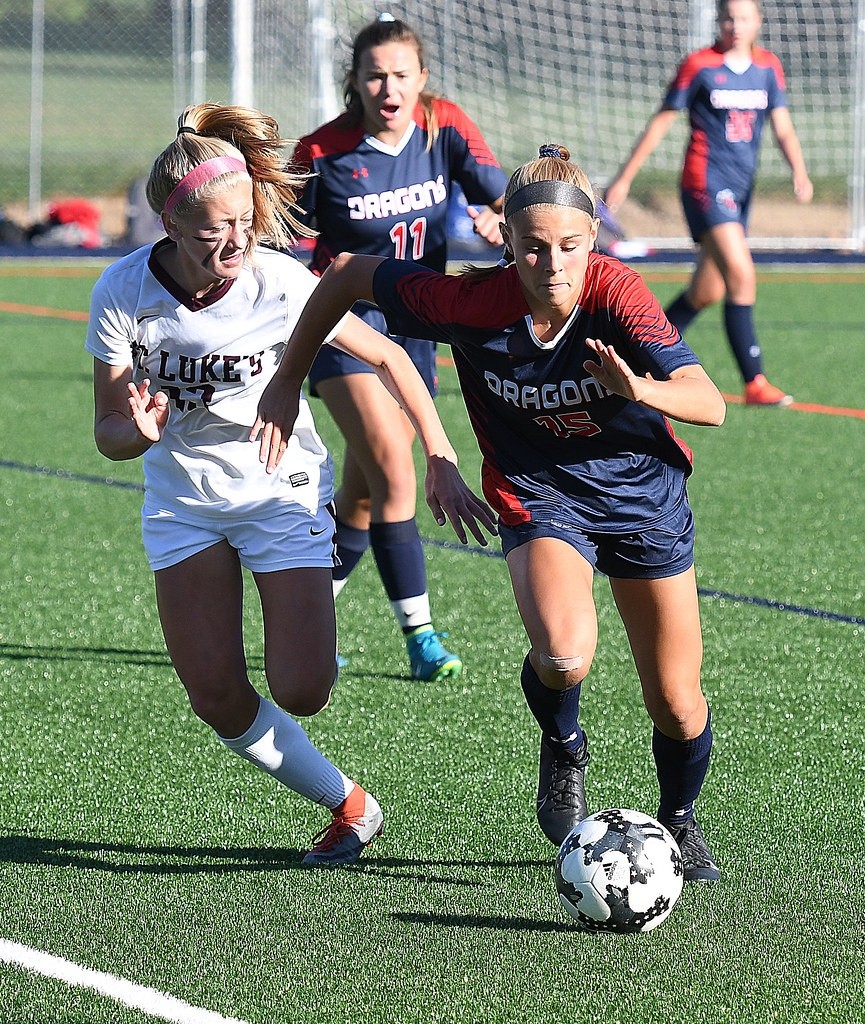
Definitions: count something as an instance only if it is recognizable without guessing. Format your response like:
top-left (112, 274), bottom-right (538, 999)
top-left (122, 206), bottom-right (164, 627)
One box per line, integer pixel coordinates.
top-left (553, 807), bottom-right (684, 934)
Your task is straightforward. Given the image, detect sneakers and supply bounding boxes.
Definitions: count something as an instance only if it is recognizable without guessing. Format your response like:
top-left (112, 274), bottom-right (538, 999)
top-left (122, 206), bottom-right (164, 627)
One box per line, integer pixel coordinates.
top-left (302, 793), bottom-right (383, 865)
top-left (535, 729), bottom-right (590, 847)
top-left (745, 373), bottom-right (793, 406)
top-left (407, 629), bottom-right (462, 683)
top-left (655, 804), bottom-right (720, 880)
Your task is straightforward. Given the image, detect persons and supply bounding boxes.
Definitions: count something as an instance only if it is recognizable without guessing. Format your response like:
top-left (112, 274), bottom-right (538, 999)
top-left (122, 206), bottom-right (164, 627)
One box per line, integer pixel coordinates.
top-left (247, 144), bottom-right (725, 886)
top-left (603, 0), bottom-right (815, 406)
top-left (84, 102), bottom-right (498, 864)
top-left (280, 16), bottom-right (508, 683)
